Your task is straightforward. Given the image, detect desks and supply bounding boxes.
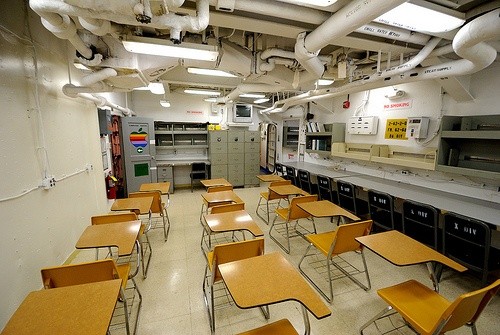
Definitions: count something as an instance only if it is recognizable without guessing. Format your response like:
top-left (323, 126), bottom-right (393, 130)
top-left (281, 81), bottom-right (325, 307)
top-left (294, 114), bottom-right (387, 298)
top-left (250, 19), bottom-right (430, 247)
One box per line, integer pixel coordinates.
top-left (0, 279), bottom-right (123, 335)
top-left (156, 160), bottom-right (211, 193)
top-left (139, 182), bottom-right (171, 209)
top-left (200, 173), bottom-right (469, 335)
top-left (111, 196), bottom-right (153, 231)
top-left (75, 221), bottom-right (141, 278)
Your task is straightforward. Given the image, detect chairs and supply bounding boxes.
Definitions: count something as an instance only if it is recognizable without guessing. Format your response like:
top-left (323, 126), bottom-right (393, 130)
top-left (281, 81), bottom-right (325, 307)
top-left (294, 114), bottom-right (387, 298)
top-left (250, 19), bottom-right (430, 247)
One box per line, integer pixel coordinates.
top-left (296, 168), bottom-right (317, 194)
top-left (286, 166), bottom-right (301, 188)
top-left (274, 163), bottom-right (292, 179)
top-left (189, 161), bottom-right (208, 192)
top-left (41, 179), bottom-right (500, 335)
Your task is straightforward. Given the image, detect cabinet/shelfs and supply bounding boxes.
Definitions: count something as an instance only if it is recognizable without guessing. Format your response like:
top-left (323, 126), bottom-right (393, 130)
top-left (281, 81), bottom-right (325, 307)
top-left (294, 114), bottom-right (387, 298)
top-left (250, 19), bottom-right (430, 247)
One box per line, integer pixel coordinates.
top-left (305, 122), bottom-right (346, 156)
top-left (154, 121), bottom-right (208, 148)
top-left (209, 127), bottom-right (261, 187)
top-left (158, 167), bottom-right (174, 193)
top-left (434, 114), bottom-right (500, 180)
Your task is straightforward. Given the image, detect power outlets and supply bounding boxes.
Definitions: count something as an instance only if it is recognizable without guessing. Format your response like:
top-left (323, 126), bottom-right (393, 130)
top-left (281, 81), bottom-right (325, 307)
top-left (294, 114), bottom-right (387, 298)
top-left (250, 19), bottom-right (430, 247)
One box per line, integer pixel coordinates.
top-left (88, 163), bottom-right (94, 173)
top-left (42, 176), bottom-right (57, 190)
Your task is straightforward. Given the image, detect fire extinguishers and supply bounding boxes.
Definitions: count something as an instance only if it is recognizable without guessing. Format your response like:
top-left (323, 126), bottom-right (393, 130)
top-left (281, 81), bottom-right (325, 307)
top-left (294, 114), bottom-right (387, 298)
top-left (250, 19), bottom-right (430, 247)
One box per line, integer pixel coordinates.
top-left (105, 171), bottom-right (116, 199)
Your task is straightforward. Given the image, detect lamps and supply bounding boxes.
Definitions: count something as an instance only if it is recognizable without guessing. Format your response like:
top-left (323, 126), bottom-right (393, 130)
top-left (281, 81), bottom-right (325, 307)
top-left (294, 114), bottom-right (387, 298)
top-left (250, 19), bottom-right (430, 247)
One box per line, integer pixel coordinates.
top-left (384, 90), bottom-right (405, 100)
top-left (315, 77), bottom-right (334, 86)
top-left (121, 34), bottom-right (219, 63)
top-left (160, 95), bottom-right (171, 108)
top-left (183, 85), bottom-right (222, 97)
